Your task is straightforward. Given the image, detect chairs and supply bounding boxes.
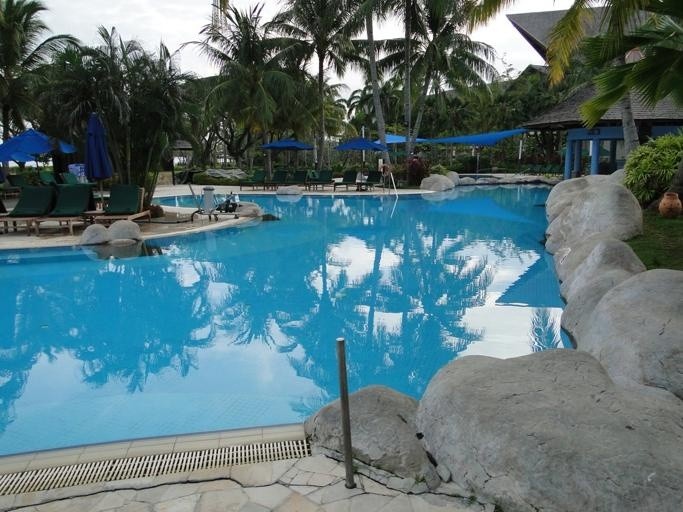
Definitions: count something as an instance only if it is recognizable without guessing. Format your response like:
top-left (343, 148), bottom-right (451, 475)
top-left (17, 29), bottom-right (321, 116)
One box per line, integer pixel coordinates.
top-left (334, 170), bottom-right (357, 191)
top-left (94, 184), bottom-right (151, 229)
top-left (357, 170), bottom-right (382, 192)
top-left (238, 169), bottom-right (332, 191)
top-left (0, 184), bottom-right (56, 236)
top-left (497, 162), bottom-right (563, 177)
top-left (35, 182), bottom-right (93, 237)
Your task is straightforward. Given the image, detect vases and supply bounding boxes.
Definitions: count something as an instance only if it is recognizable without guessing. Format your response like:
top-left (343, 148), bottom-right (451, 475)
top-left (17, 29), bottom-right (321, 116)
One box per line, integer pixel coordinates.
top-left (658, 192), bottom-right (681, 219)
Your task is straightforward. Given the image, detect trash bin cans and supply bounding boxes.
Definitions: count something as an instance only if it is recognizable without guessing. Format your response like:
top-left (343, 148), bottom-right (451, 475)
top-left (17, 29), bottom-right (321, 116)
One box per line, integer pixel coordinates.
top-left (203, 187), bottom-right (215, 211)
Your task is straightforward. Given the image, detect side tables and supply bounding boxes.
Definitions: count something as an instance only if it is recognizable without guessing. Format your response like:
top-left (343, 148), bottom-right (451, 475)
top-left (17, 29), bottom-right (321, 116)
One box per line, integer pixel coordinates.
top-left (81, 209), bottom-right (106, 225)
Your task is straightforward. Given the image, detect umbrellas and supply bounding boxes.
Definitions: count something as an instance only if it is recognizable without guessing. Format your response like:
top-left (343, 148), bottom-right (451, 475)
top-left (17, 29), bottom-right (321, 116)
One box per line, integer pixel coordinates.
top-left (260, 138), bottom-right (314, 168)
top-left (332, 137), bottom-right (391, 183)
top-left (0, 129), bottom-right (80, 175)
top-left (84, 112), bottom-right (115, 202)
